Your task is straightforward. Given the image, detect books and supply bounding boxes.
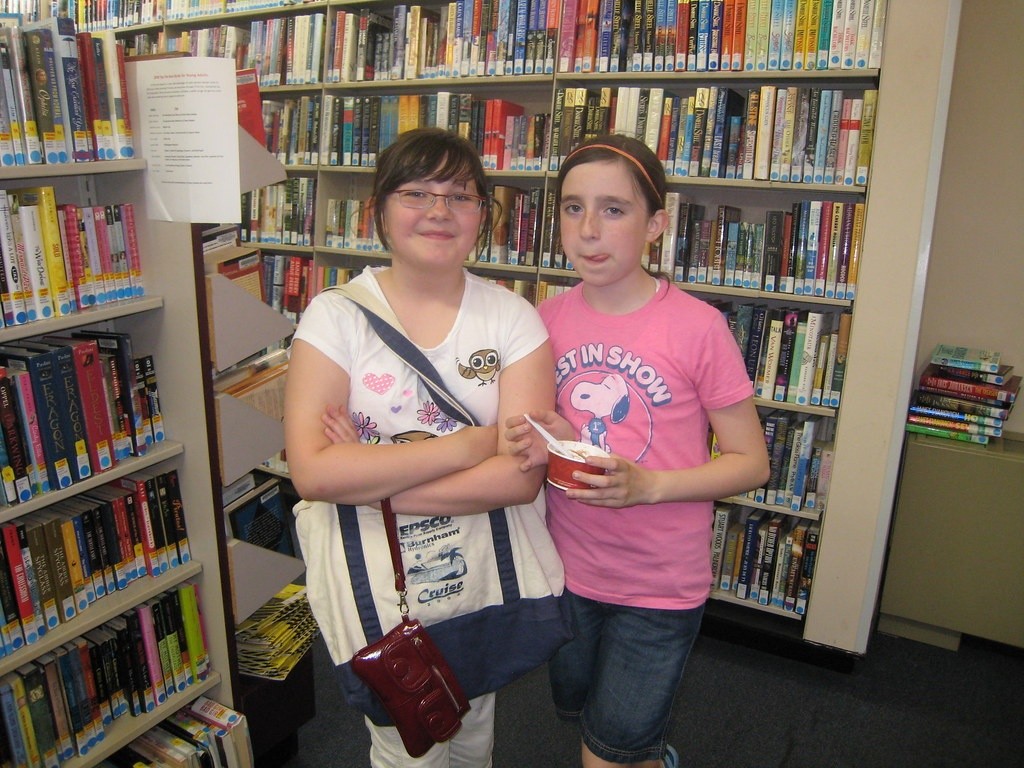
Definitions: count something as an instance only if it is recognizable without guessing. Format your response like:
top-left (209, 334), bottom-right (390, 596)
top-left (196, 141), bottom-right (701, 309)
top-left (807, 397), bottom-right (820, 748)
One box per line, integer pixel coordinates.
top-left (906, 343), bottom-right (1024, 446)
top-left (266, 0), bottom-right (886, 616)
top-left (0, 0), bottom-right (290, 768)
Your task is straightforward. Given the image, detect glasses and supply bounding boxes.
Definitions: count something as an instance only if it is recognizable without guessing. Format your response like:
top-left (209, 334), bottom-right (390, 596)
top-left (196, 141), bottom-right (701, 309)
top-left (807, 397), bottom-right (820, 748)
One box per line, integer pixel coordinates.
top-left (389, 188), bottom-right (488, 214)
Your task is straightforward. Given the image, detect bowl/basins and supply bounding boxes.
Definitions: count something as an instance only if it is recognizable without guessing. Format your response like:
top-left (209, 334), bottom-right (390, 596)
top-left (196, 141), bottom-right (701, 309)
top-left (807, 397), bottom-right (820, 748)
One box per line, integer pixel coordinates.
top-left (545, 439), bottom-right (611, 492)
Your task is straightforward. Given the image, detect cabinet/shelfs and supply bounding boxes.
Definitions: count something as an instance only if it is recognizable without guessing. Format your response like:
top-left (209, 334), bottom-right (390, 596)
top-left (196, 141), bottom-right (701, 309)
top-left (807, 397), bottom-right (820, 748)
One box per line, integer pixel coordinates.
top-left (877, 430), bottom-right (1024, 651)
top-left (125, 51), bottom-right (317, 768)
top-left (115, 0), bottom-right (963, 654)
top-left (0, 160), bottom-right (222, 768)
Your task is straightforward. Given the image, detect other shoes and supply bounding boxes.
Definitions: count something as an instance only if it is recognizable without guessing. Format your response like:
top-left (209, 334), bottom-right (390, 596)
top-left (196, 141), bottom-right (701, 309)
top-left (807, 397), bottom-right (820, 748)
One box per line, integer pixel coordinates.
top-left (661, 744), bottom-right (679, 768)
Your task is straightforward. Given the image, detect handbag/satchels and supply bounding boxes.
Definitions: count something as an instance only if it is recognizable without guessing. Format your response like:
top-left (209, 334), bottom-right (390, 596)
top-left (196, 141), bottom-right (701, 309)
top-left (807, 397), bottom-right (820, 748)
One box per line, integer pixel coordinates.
top-left (350, 496), bottom-right (471, 760)
top-left (291, 284), bottom-right (576, 727)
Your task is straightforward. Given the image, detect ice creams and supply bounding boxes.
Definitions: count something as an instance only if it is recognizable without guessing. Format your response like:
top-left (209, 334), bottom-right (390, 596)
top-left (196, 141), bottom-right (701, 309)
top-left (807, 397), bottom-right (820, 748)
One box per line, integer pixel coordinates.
top-left (547, 440), bottom-right (610, 491)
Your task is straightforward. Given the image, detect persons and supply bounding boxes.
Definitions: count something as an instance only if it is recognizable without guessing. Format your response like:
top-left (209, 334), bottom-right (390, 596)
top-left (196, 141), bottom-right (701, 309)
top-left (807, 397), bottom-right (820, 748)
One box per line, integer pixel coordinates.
top-left (284, 127), bottom-right (555, 768)
top-left (503, 134), bottom-right (769, 768)
